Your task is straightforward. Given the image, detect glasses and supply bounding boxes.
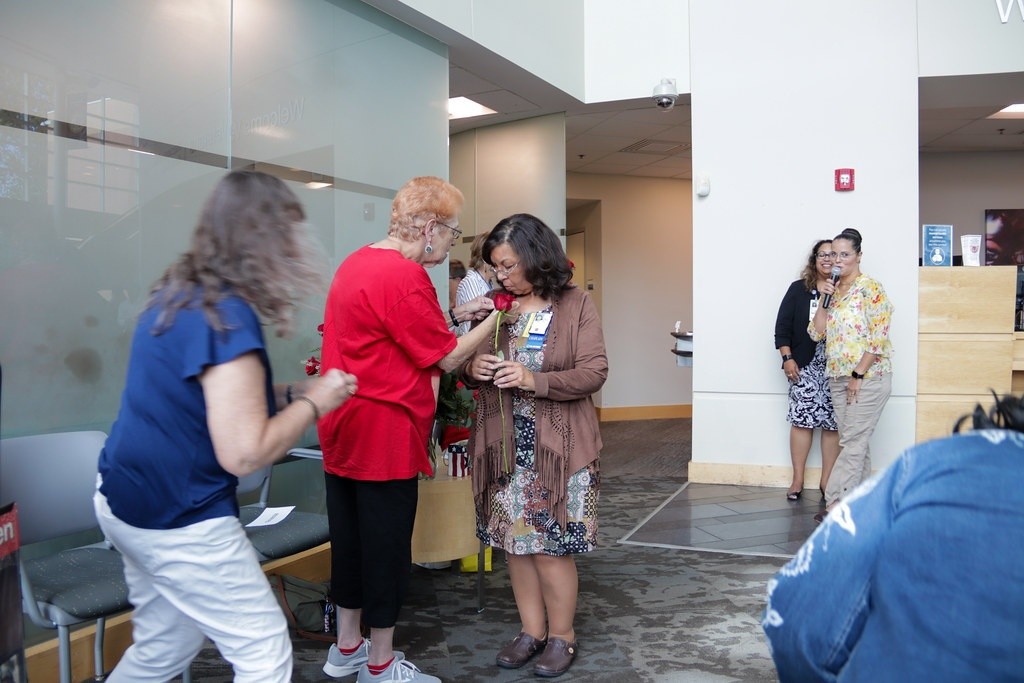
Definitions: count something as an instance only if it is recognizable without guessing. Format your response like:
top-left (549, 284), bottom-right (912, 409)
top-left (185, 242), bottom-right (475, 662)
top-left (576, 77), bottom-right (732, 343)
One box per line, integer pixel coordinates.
top-left (831, 252), bottom-right (857, 260)
top-left (815, 252), bottom-right (830, 260)
top-left (488, 260), bottom-right (521, 277)
top-left (436, 221), bottom-right (463, 239)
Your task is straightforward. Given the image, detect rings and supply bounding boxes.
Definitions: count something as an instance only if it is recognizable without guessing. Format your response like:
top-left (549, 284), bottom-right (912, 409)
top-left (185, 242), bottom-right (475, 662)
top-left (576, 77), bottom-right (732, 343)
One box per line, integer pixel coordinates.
top-left (851, 393), bottom-right (855, 395)
top-left (787, 374), bottom-right (793, 376)
top-left (348, 384), bottom-right (356, 396)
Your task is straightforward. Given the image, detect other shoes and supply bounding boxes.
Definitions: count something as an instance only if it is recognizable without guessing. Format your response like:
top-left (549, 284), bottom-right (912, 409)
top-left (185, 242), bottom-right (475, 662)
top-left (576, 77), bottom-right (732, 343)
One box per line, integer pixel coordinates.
top-left (787, 486), bottom-right (803, 500)
top-left (814, 511), bottom-right (828, 524)
top-left (820, 486), bottom-right (824, 496)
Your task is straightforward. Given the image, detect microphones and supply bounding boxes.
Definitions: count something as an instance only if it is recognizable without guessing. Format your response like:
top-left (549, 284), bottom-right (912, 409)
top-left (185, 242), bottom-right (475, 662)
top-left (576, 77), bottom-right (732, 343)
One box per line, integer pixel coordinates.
top-left (822, 267), bottom-right (840, 309)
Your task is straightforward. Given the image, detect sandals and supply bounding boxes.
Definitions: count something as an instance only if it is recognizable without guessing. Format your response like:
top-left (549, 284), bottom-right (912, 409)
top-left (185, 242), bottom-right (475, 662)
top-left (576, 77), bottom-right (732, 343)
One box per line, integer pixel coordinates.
top-left (496, 628), bottom-right (548, 668)
top-left (533, 632), bottom-right (579, 677)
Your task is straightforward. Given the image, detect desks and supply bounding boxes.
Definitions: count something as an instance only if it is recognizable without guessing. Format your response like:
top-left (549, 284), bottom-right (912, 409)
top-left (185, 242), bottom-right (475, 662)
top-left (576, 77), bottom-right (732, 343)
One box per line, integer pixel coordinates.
top-left (412, 451), bottom-right (488, 613)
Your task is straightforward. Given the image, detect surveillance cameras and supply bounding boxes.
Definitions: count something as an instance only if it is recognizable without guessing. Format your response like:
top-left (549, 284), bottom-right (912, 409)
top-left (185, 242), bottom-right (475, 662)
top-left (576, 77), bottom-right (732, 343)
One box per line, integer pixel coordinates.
top-left (651, 85), bottom-right (679, 110)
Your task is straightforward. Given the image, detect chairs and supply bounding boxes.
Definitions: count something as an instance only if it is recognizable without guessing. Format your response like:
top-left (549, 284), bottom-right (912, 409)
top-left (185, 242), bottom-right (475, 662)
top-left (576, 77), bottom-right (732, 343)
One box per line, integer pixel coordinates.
top-left (0, 428), bottom-right (328, 683)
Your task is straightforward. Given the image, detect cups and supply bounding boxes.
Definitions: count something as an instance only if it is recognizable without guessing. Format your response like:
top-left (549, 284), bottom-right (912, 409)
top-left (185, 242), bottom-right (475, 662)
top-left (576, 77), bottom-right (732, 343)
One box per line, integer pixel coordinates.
top-left (447, 444), bottom-right (470, 477)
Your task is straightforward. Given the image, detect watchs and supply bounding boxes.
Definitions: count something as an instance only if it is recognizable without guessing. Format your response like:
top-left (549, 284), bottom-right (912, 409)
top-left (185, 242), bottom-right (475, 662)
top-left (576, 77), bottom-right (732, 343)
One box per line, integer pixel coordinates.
top-left (782, 354), bottom-right (793, 362)
top-left (851, 371), bottom-right (864, 379)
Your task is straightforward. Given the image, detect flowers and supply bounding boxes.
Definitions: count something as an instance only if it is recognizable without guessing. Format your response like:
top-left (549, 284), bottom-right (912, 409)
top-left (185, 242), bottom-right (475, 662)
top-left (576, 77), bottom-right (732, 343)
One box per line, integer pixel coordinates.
top-left (300, 323), bottom-right (324, 376)
top-left (567, 258), bottom-right (576, 272)
top-left (491, 290), bottom-right (517, 475)
top-left (435, 369), bottom-right (480, 429)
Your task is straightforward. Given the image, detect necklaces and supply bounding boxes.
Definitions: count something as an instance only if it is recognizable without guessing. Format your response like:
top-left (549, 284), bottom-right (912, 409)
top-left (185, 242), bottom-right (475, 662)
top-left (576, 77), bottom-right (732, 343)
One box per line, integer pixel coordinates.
top-left (839, 283), bottom-right (853, 286)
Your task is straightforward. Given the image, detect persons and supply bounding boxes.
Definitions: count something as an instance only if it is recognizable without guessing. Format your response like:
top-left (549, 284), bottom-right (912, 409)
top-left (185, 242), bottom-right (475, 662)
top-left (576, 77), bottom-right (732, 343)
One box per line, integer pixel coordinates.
top-left (762, 389), bottom-right (1024, 683)
top-left (316, 176), bottom-right (520, 683)
top-left (774, 240), bottom-right (844, 501)
top-left (93, 169), bottom-right (358, 683)
top-left (807, 227), bottom-right (894, 523)
top-left (454, 212), bottom-right (608, 677)
top-left (414, 231), bottom-right (496, 571)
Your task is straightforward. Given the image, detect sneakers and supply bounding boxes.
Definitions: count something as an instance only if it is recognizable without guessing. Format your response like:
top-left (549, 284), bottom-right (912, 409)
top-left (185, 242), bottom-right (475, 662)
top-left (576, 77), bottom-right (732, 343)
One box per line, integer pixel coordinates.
top-left (323, 637), bottom-right (405, 677)
top-left (356, 656), bottom-right (441, 683)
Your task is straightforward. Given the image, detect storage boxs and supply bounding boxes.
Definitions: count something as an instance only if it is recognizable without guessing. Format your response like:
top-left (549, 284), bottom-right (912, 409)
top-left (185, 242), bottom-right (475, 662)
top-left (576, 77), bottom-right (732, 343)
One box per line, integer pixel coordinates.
top-left (960, 234), bottom-right (981, 267)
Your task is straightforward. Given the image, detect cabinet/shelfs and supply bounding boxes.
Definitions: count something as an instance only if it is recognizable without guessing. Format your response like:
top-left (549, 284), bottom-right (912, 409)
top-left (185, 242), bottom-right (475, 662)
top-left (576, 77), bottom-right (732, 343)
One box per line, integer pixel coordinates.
top-left (669, 331), bottom-right (693, 367)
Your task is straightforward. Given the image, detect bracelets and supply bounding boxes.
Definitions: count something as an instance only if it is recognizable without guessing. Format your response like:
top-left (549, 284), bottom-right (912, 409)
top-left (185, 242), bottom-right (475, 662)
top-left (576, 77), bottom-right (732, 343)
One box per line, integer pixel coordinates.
top-left (285, 384), bottom-right (293, 404)
top-left (296, 396), bottom-right (319, 420)
top-left (448, 309), bottom-right (460, 327)
